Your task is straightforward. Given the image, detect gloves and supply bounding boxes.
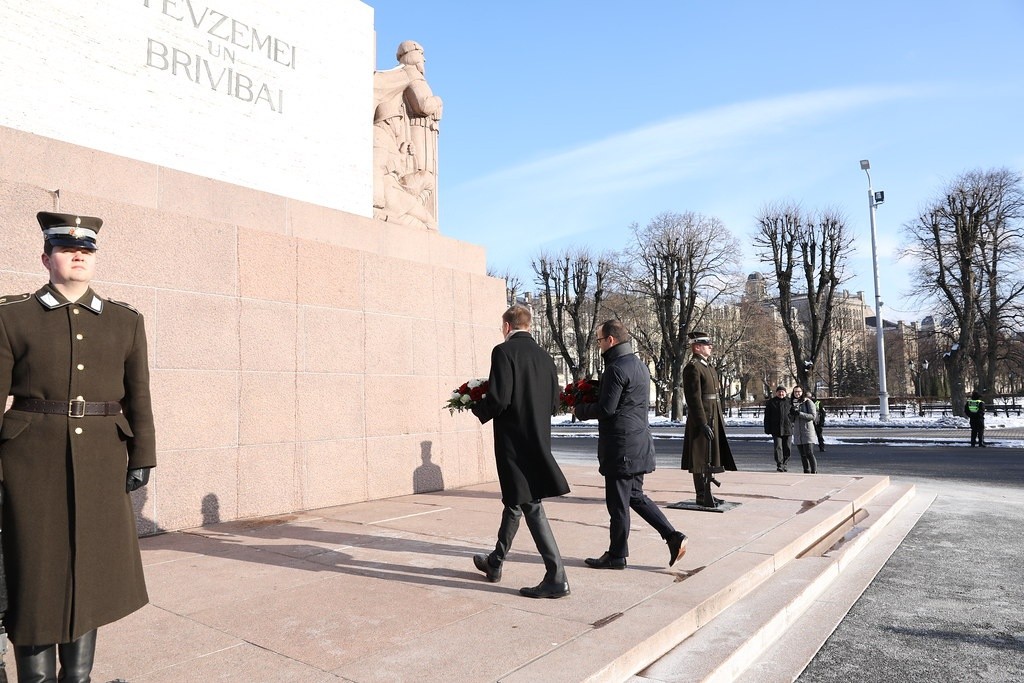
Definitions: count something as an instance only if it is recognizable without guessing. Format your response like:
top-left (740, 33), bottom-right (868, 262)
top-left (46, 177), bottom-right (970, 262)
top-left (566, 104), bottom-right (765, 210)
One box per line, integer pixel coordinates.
top-left (790, 406), bottom-right (799, 416)
top-left (126, 466), bottom-right (150, 494)
top-left (703, 423), bottom-right (715, 440)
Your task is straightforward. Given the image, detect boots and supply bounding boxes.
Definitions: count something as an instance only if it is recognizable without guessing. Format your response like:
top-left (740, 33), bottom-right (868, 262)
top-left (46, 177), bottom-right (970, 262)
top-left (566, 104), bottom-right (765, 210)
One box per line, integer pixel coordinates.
top-left (692, 472), bottom-right (720, 507)
top-left (712, 495), bottom-right (726, 505)
top-left (13, 641), bottom-right (57, 683)
top-left (57, 627), bottom-right (97, 683)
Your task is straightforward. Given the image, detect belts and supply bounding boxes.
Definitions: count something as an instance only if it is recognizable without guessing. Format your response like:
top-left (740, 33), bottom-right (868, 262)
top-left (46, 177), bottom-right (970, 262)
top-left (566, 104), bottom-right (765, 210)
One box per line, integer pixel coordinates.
top-left (10, 396), bottom-right (121, 418)
top-left (702, 393), bottom-right (720, 399)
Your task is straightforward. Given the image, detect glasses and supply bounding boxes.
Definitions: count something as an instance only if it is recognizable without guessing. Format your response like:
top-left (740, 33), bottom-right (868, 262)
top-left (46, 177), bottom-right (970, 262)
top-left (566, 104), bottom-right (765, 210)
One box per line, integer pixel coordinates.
top-left (596, 335), bottom-right (614, 344)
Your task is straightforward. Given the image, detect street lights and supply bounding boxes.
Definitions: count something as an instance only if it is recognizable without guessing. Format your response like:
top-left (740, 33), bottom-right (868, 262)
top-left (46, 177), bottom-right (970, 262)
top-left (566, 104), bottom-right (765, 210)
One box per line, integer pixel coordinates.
top-left (908, 359), bottom-right (929, 416)
top-left (859, 160), bottom-right (890, 420)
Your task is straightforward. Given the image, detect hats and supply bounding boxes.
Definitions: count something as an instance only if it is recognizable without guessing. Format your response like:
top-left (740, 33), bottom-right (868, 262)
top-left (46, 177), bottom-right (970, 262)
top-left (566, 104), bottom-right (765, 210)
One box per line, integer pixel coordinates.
top-left (36, 211), bottom-right (103, 249)
top-left (687, 331), bottom-right (713, 345)
top-left (776, 386), bottom-right (786, 393)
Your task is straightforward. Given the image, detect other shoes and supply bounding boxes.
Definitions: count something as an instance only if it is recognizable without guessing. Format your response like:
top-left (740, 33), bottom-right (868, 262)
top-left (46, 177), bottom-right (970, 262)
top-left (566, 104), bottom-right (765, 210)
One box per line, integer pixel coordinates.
top-left (784, 466), bottom-right (787, 472)
top-left (971, 444), bottom-right (975, 447)
top-left (820, 448), bottom-right (825, 452)
top-left (979, 444), bottom-right (986, 448)
top-left (777, 467), bottom-right (783, 472)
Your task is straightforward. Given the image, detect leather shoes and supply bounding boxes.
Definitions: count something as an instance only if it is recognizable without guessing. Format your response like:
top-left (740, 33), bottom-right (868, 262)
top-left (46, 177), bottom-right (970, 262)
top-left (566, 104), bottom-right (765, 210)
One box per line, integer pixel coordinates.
top-left (584, 551), bottom-right (626, 570)
top-left (519, 580), bottom-right (570, 598)
top-left (667, 530), bottom-right (688, 567)
top-left (473, 554), bottom-right (502, 583)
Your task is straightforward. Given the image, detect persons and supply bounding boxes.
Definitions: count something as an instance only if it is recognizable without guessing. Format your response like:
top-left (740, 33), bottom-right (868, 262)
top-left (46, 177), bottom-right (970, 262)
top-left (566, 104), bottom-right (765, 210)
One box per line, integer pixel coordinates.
top-left (680, 331), bottom-right (738, 506)
top-left (470, 306), bottom-right (571, 600)
top-left (763, 385), bottom-right (793, 472)
top-left (806, 390), bottom-right (826, 452)
top-left (571, 320), bottom-right (688, 570)
top-left (791, 386), bottom-right (818, 474)
top-left (375, 40), bottom-right (442, 231)
top-left (965, 392), bottom-right (988, 447)
top-left (0, 211), bottom-right (157, 683)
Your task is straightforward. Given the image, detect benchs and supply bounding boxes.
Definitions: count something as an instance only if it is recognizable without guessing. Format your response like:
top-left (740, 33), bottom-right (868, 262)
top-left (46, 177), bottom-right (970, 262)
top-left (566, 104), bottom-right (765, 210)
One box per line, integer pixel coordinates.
top-left (984, 404), bottom-right (1022, 416)
top-left (864, 405), bottom-right (906, 417)
top-left (921, 405), bottom-right (952, 416)
top-left (738, 407), bottom-right (766, 417)
top-left (824, 406), bottom-right (862, 417)
top-left (722, 407), bottom-right (728, 418)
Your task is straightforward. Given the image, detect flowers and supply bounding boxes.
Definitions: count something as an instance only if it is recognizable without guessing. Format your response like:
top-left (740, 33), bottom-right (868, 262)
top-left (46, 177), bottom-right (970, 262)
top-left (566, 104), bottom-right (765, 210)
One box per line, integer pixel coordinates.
top-left (444, 377), bottom-right (490, 416)
top-left (553, 378), bottom-right (600, 423)
